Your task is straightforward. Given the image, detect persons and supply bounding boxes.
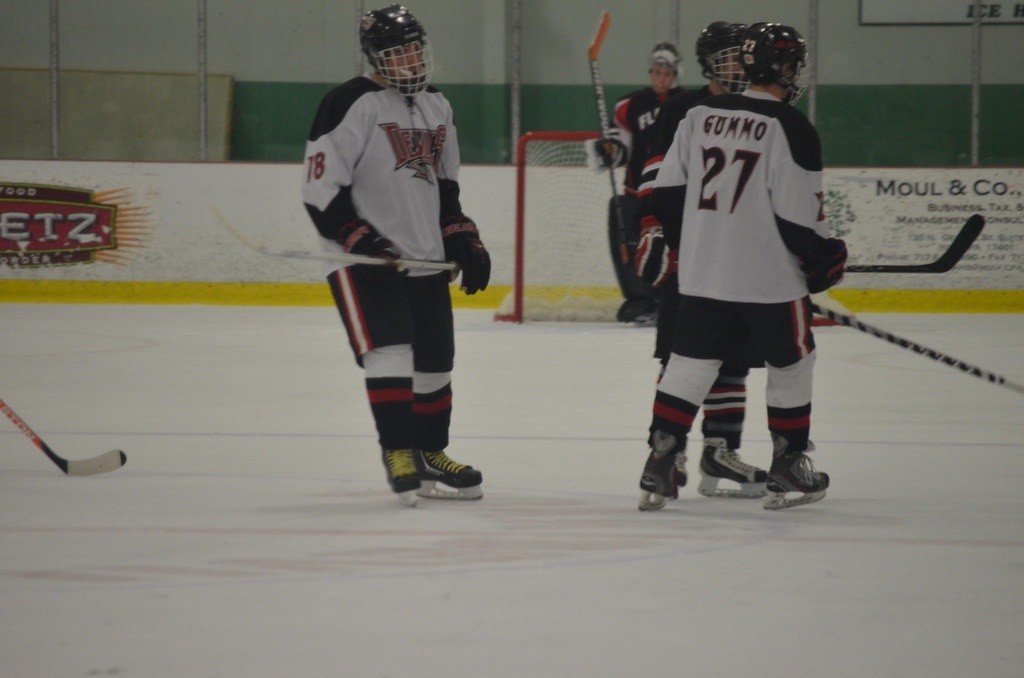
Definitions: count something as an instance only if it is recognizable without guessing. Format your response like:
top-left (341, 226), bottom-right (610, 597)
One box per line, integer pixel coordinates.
top-left (633, 22), bottom-right (769, 496)
top-left (639, 23), bottom-right (846, 510)
top-left (303, 4), bottom-right (493, 504)
top-left (592, 43), bottom-right (680, 320)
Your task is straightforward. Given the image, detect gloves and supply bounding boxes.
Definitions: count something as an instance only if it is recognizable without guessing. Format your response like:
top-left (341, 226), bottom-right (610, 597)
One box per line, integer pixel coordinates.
top-left (440, 213), bottom-right (491, 295)
top-left (596, 139), bottom-right (628, 168)
top-left (338, 218), bottom-right (409, 278)
top-left (633, 216), bottom-right (678, 287)
top-left (802, 235), bottom-right (847, 293)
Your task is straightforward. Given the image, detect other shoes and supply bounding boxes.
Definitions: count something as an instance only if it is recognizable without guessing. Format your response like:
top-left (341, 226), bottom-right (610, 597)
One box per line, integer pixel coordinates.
top-left (633, 308), bottom-right (659, 327)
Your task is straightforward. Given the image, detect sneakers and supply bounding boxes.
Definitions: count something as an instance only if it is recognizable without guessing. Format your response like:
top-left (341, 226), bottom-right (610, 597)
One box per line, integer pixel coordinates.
top-left (698, 434), bottom-right (768, 498)
top-left (410, 447), bottom-right (484, 500)
top-left (638, 425), bottom-right (678, 510)
top-left (762, 433), bottom-right (829, 509)
top-left (382, 447), bottom-right (421, 506)
top-left (672, 432), bottom-right (687, 494)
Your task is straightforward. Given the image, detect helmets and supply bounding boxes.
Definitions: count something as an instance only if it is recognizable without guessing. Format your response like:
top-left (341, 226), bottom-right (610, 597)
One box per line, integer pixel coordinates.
top-left (360, 4), bottom-right (435, 102)
top-left (738, 21), bottom-right (807, 86)
top-left (697, 20), bottom-right (749, 94)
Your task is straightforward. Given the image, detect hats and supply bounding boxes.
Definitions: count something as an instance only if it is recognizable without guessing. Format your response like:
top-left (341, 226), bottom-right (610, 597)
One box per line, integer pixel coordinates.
top-left (648, 44), bottom-right (679, 66)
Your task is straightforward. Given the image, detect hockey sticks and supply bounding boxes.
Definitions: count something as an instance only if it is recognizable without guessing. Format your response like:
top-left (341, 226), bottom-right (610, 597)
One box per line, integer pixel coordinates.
top-left (842, 213), bottom-right (987, 274)
top-left (0, 400), bottom-right (127, 475)
top-left (810, 301), bottom-right (1024, 393)
top-left (586, 11), bottom-right (630, 265)
top-left (212, 202), bottom-right (457, 271)
